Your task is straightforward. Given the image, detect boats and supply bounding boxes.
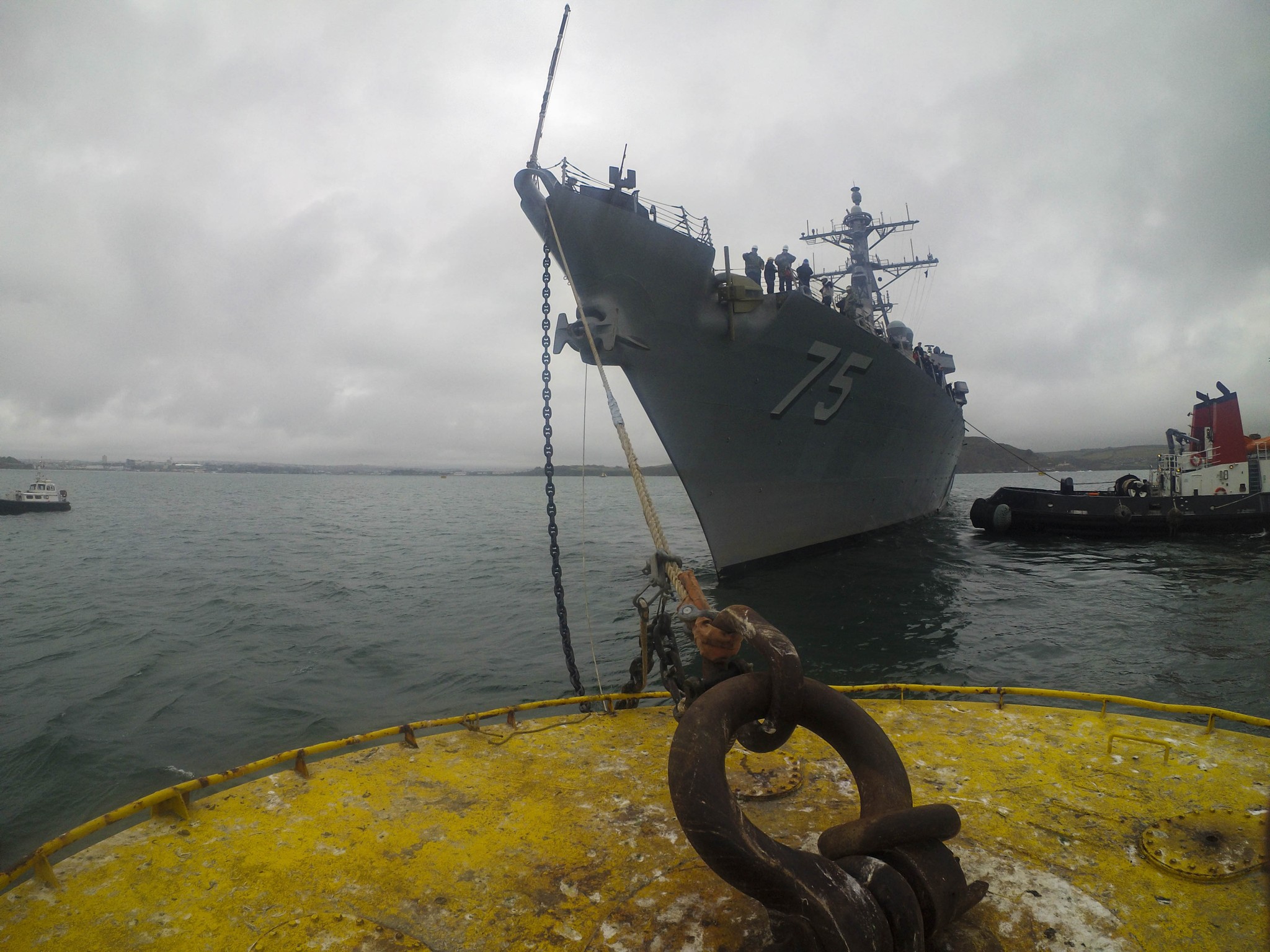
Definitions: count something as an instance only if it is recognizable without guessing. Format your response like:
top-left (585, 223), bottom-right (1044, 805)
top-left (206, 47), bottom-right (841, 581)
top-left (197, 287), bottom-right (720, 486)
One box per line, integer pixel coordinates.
top-left (968, 381), bottom-right (1270, 542)
top-left (0, 466), bottom-right (71, 516)
top-left (511, 3), bottom-right (971, 578)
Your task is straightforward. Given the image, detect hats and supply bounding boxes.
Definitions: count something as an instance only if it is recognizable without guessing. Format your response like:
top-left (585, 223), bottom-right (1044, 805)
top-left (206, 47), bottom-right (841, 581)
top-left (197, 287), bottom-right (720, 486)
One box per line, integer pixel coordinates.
top-left (803, 259), bottom-right (808, 262)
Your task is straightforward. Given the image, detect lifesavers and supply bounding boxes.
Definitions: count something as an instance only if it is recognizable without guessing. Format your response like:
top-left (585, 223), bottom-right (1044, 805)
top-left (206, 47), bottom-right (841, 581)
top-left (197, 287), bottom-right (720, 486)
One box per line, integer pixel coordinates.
top-left (1215, 487), bottom-right (1227, 495)
top-left (1190, 453), bottom-right (1202, 466)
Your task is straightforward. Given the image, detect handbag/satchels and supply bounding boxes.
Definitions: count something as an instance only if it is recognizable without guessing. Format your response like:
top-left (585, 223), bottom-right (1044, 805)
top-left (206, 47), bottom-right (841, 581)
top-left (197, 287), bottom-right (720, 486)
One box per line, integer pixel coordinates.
top-left (752, 267), bottom-right (760, 276)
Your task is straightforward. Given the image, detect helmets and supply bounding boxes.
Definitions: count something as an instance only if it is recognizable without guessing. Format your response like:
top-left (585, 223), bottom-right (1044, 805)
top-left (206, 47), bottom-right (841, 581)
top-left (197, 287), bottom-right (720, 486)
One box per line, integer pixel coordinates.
top-left (782, 245), bottom-right (788, 250)
top-left (751, 245), bottom-right (758, 249)
top-left (821, 276), bottom-right (827, 280)
top-left (841, 294), bottom-right (846, 297)
top-left (844, 292), bottom-right (848, 295)
top-left (846, 286), bottom-right (851, 290)
top-left (767, 257), bottom-right (774, 261)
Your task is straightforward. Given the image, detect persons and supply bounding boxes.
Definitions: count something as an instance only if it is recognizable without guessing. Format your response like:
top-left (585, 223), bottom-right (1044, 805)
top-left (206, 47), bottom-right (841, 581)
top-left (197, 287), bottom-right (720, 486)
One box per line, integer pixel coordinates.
top-left (796, 259), bottom-right (813, 288)
top-left (836, 294), bottom-right (846, 316)
top-left (764, 256), bottom-right (778, 294)
top-left (15, 490), bottom-right (22, 501)
top-left (775, 244), bottom-right (796, 292)
top-left (819, 275), bottom-right (833, 308)
top-left (742, 244), bottom-right (765, 286)
top-left (844, 285), bottom-right (858, 321)
top-left (946, 381), bottom-right (953, 396)
top-left (913, 342), bottom-right (941, 387)
